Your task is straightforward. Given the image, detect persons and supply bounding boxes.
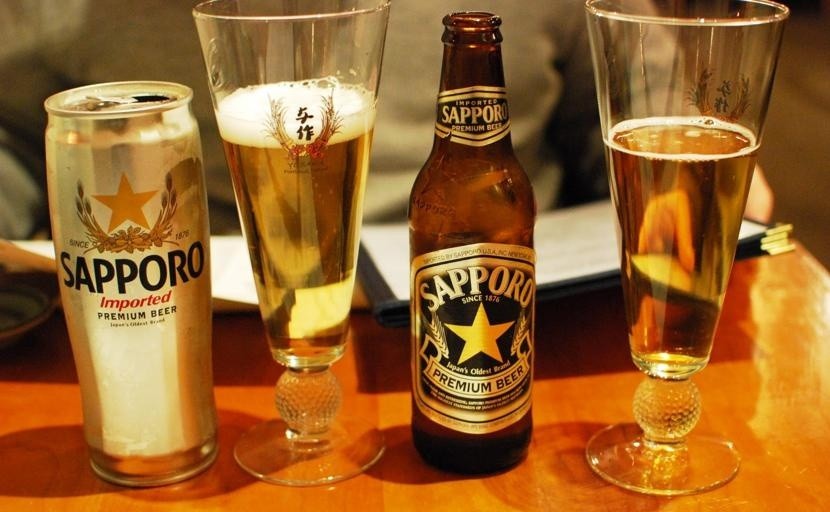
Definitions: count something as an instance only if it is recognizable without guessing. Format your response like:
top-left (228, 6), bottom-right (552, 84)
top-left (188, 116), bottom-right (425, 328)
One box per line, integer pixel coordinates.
top-left (0, 1), bottom-right (779, 253)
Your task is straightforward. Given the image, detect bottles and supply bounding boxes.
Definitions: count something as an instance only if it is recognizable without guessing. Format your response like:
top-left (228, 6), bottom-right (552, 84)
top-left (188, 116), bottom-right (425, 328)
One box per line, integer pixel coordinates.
top-left (407, 9), bottom-right (537, 475)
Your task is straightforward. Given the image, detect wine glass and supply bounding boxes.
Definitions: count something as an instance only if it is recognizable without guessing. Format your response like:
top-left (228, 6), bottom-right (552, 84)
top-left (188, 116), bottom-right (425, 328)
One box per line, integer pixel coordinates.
top-left (586, 0), bottom-right (789, 497)
top-left (194, 0), bottom-right (393, 485)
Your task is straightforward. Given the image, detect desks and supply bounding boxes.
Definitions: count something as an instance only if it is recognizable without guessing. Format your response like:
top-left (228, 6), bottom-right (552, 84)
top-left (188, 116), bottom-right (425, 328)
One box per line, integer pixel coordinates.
top-left (0, 228), bottom-right (830, 512)
